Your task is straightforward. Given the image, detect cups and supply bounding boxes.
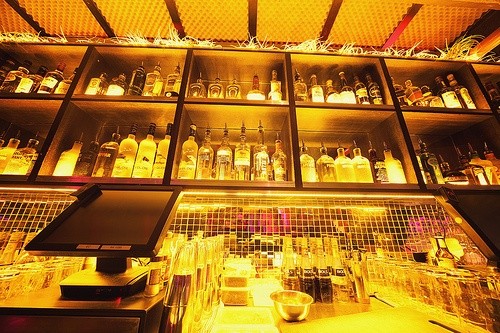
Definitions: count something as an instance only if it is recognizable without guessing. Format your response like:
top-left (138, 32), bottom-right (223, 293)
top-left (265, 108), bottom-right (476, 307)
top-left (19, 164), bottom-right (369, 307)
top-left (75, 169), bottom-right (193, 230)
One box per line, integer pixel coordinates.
top-left (0, 246), bottom-right (96, 301)
top-left (355, 248), bottom-right (500, 329)
top-left (149, 231), bottom-right (229, 333)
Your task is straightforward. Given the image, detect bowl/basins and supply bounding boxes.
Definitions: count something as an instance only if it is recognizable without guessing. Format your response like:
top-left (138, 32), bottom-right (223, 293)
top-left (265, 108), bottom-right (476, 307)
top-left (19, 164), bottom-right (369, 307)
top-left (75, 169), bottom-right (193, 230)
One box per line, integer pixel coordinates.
top-left (268, 289), bottom-right (313, 321)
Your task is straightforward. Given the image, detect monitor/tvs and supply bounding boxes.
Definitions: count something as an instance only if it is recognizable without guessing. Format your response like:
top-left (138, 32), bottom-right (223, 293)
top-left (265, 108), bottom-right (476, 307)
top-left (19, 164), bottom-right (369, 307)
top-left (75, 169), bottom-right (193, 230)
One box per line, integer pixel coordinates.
top-left (433, 185), bottom-right (500, 266)
top-left (25, 184), bottom-right (184, 302)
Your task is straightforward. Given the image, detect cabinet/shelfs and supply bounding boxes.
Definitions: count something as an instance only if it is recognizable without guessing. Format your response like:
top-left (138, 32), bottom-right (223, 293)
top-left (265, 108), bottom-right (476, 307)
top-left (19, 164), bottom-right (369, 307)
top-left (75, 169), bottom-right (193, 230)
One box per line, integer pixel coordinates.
top-left (0, 39), bottom-right (500, 193)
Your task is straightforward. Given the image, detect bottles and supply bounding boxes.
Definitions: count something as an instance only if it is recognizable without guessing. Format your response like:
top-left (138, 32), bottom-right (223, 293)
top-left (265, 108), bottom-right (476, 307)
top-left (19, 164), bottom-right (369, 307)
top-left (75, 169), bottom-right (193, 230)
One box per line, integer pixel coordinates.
top-left (1, 57), bottom-right (500, 186)
top-left (278, 232), bottom-right (373, 307)
